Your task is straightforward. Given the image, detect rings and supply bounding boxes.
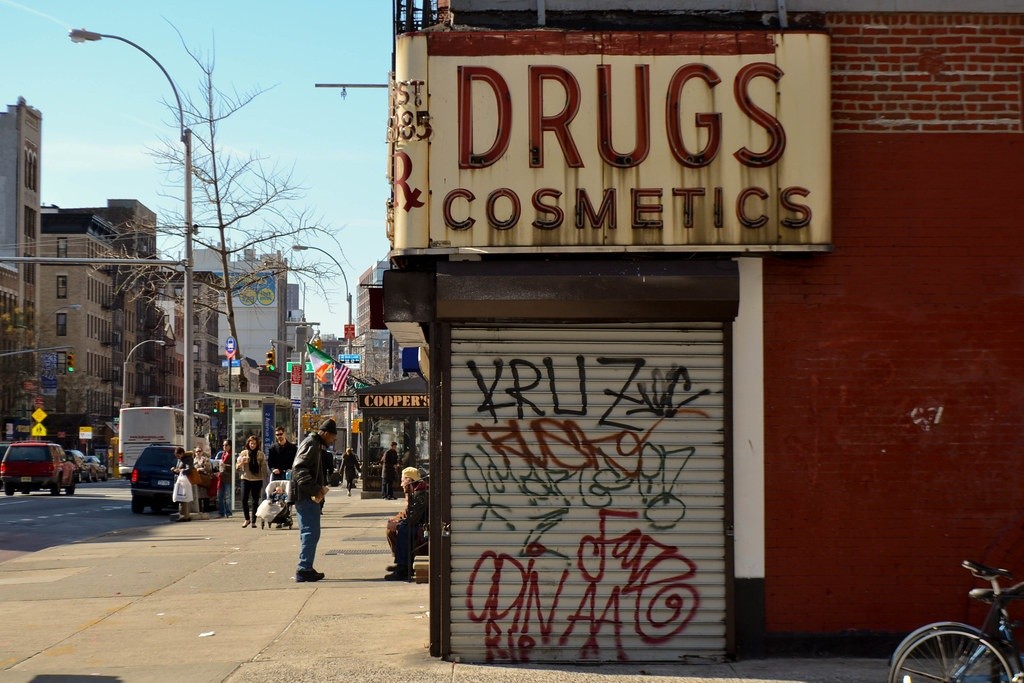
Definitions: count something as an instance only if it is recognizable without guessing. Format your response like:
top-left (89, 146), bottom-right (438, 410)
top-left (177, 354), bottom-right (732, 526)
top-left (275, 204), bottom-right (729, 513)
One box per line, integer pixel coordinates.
top-left (321, 497), bottom-right (323, 500)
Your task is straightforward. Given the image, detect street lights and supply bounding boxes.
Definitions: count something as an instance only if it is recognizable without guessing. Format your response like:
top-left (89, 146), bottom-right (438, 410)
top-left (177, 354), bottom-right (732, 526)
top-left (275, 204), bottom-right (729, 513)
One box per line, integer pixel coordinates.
top-left (70, 28), bottom-right (193, 452)
top-left (293, 245), bottom-right (352, 449)
top-left (122, 340), bottom-right (166, 406)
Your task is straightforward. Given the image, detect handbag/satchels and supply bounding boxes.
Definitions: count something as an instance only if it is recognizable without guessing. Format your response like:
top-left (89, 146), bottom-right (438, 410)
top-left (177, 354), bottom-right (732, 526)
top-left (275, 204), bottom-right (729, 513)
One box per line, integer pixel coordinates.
top-left (197, 469), bottom-right (210, 488)
top-left (173, 469), bottom-right (193, 502)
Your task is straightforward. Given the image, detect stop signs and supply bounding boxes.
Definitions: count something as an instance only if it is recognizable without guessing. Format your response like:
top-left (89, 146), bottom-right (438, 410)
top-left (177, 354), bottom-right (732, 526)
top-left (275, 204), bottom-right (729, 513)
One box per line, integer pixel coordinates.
top-left (34, 398), bottom-right (44, 408)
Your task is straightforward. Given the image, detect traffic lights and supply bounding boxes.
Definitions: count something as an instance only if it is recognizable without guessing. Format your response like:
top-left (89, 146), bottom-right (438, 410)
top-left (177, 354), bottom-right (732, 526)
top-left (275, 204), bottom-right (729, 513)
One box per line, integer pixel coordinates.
top-left (220, 402), bottom-right (224, 413)
top-left (214, 401), bottom-right (218, 412)
top-left (266, 351), bottom-right (275, 372)
top-left (67, 354), bottom-right (75, 372)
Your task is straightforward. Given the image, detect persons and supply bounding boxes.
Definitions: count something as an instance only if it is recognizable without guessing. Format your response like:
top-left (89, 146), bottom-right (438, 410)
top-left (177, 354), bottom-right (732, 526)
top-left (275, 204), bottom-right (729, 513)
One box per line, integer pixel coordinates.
top-left (170, 438), bottom-right (232, 522)
top-left (235, 435), bottom-right (268, 528)
top-left (291, 419), bottom-right (338, 582)
top-left (381, 441), bottom-right (399, 499)
top-left (269, 486), bottom-right (288, 508)
top-left (384, 466), bottom-right (430, 580)
top-left (339, 448), bottom-right (362, 497)
top-left (268, 426), bottom-right (298, 528)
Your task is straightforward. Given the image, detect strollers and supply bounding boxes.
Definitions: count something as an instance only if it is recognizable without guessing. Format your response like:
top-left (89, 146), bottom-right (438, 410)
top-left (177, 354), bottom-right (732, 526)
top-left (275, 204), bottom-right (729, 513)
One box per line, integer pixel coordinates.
top-left (262, 470), bottom-right (294, 529)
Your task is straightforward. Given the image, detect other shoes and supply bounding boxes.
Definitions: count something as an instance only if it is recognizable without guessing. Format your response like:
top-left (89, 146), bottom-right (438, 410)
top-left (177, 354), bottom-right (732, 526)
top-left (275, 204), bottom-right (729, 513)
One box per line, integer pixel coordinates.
top-left (242, 520), bottom-right (250, 528)
top-left (384, 565), bottom-right (414, 581)
top-left (295, 568), bottom-right (325, 583)
top-left (252, 525), bottom-right (257, 528)
top-left (176, 517), bottom-right (191, 522)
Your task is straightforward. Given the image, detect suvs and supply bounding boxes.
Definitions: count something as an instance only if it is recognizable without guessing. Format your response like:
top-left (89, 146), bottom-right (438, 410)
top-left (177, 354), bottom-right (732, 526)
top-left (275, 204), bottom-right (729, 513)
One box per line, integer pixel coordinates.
top-left (64, 449), bottom-right (91, 483)
top-left (130, 443), bottom-right (185, 513)
top-left (1, 441), bottom-right (76, 496)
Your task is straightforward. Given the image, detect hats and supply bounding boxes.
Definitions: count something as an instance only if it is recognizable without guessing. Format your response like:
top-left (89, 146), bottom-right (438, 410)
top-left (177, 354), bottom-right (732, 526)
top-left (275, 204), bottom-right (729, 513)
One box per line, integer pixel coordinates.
top-left (319, 418), bottom-right (338, 434)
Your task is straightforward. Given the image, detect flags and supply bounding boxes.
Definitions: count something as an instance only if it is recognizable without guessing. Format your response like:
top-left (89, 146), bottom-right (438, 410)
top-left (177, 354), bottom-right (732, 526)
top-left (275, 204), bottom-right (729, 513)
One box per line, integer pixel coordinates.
top-left (333, 359), bottom-right (351, 392)
top-left (305, 341), bottom-right (334, 382)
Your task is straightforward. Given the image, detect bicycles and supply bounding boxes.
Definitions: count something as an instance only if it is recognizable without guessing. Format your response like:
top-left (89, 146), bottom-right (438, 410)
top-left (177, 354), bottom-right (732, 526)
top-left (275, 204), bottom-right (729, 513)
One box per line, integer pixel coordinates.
top-left (968, 581), bottom-right (1024, 683)
top-left (887, 560), bottom-right (1024, 683)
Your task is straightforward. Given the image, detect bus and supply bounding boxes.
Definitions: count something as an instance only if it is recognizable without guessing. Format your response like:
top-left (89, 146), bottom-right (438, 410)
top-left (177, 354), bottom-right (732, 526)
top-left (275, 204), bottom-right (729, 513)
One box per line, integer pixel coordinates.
top-left (118, 406), bottom-right (211, 480)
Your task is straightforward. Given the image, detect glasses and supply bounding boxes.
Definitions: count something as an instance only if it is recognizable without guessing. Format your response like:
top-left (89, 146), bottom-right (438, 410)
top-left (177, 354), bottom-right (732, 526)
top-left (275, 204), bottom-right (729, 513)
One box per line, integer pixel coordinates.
top-left (275, 433), bottom-right (283, 436)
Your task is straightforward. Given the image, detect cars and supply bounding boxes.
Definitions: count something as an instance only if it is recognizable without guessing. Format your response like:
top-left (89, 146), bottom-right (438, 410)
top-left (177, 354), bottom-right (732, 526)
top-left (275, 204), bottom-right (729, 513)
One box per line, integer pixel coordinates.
top-left (211, 451), bottom-right (224, 471)
top-left (84, 456), bottom-right (108, 482)
